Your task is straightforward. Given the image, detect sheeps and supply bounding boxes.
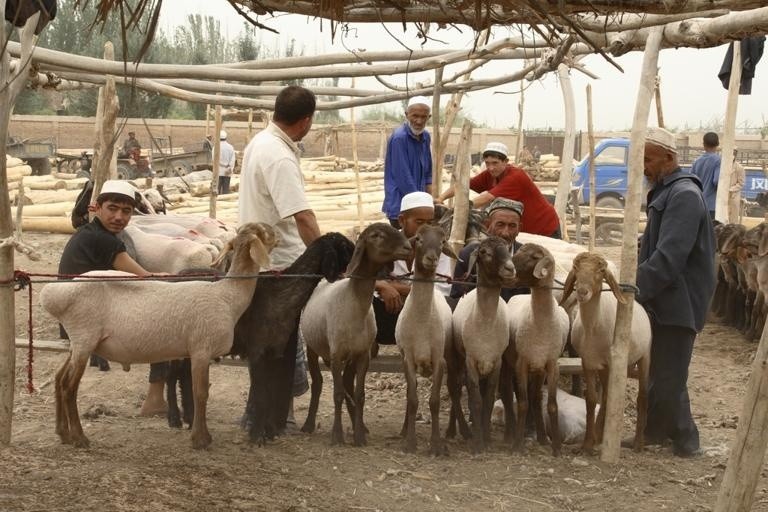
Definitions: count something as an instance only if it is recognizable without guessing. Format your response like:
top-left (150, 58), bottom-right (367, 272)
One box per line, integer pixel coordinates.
top-left (494, 383), bottom-right (600, 444)
top-left (116, 186), bottom-right (237, 275)
top-left (710, 218), bottom-right (768, 341)
top-left (503, 242), bottom-right (569, 455)
top-left (558, 252), bottom-right (652, 457)
top-left (165, 232), bottom-right (355, 448)
top-left (444, 235), bottom-right (517, 455)
top-left (516, 231), bottom-right (620, 397)
top-left (395, 223), bottom-right (465, 459)
top-left (40, 223), bottom-right (283, 450)
top-left (299, 222), bottom-right (413, 448)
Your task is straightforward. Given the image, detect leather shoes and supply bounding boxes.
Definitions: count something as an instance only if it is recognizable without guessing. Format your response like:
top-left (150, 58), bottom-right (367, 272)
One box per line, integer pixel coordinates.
top-left (620, 434), bottom-right (656, 446)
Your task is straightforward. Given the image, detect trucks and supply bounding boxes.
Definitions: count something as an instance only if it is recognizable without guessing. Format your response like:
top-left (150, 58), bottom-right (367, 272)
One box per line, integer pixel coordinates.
top-left (571, 138), bottom-right (767, 226)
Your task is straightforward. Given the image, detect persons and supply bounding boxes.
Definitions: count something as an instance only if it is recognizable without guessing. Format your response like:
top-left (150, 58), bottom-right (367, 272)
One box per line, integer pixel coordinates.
top-left (238, 86), bottom-right (323, 432)
top-left (730, 147), bottom-right (745, 224)
top-left (56, 180), bottom-right (171, 417)
top-left (619, 125), bottom-right (715, 458)
top-left (371, 192), bottom-right (433, 347)
top-left (519, 144), bottom-right (532, 163)
top-left (212, 130), bottom-right (235, 195)
top-left (202, 134), bottom-right (213, 151)
top-left (122, 130), bottom-right (142, 155)
top-left (689, 131), bottom-right (720, 219)
top-left (450, 196), bottom-right (526, 302)
top-left (381, 96), bottom-right (432, 231)
top-left (532, 145), bottom-right (541, 162)
top-left (434, 142), bottom-right (563, 240)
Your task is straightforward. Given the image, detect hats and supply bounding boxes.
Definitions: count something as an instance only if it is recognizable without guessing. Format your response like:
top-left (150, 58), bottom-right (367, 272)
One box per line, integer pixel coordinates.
top-left (407, 95), bottom-right (431, 109)
top-left (97, 178), bottom-right (137, 202)
top-left (645, 126), bottom-right (680, 154)
top-left (481, 141), bottom-right (510, 160)
top-left (220, 131), bottom-right (227, 140)
top-left (398, 190), bottom-right (437, 211)
top-left (486, 195), bottom-right (526, 217)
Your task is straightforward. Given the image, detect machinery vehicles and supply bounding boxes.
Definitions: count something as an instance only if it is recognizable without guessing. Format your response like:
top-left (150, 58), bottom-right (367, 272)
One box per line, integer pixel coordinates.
top-left (4, 136), bottom-right (51, 175)
top-left (56, 136), bottom-right (215, 181)
